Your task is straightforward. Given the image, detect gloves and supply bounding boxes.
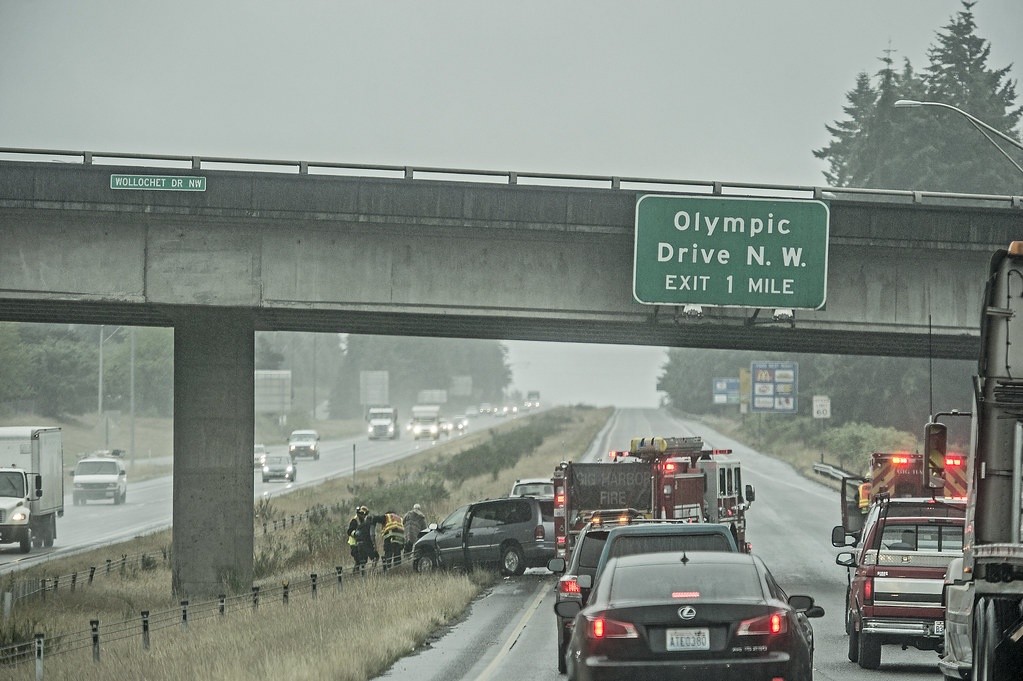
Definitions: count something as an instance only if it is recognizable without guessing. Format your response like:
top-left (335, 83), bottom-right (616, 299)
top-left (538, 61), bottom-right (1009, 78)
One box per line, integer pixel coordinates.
top-left (354, 531), bottom-right (361, 536)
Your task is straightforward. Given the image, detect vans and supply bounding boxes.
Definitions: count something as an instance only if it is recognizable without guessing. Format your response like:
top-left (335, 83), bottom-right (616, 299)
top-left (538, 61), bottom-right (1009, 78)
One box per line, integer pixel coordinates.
top-left (593, 522), bottom-right (739, 587)
top-left (68, 455), bottom-right (127, 506)
top-left (287, 429), bottom-right (321, 461)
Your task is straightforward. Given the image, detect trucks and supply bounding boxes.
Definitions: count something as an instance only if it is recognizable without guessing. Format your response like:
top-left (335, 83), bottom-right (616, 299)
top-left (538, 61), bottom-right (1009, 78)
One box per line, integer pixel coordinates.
top-left (364, 407), bottom-right (399, 441)
top-left (550, 434), bottom-right (756, 595)
top-left (0, 425), bottom-right (64, 555)
top-left (841, 450), bottom-right (968, 535)
top-left (411, 405), bottom-right (441, 440)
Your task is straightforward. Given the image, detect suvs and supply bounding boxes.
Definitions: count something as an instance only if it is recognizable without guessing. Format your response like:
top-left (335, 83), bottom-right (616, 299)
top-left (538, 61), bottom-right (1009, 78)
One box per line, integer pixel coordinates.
top-left (547, 519), bottom-right (691, 673)
top-left (413, 491), bottom-right (556, 576)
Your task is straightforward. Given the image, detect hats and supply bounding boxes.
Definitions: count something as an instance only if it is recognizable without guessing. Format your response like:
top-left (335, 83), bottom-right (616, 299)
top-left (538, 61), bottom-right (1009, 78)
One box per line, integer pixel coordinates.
top-left (413, 504), bottom-right (421, 510)
top-left (357, 506), bottom-right (369, 513)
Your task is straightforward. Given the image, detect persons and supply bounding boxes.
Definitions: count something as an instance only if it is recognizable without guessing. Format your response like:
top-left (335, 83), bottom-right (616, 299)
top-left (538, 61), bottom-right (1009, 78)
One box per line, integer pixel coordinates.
top-left (371, 511), bottom-right (405, 569)
top-left (355, 516), bottom-right (379, 570)
top-left (403, 504), bottom-right (427, 557)
top-left (854, 473), bottom-right (873, 508)
top-left (347, 506), bottom-right (369, 572)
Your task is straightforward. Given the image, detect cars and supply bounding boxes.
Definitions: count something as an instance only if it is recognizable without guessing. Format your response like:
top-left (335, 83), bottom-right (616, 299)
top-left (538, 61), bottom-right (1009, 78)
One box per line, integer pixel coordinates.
top-left (777, 584), bottom-right (825, 649)
top-left (831, 492), bottom-right (968, 669)
top-left (440, 389), bottom-right (542, 437)
top-left (262, 454), bottom-right (298, 482)
top-left (254, 445), bottom-right (270, 467)
top-left (563, 550), bottom-right (815, 681)
top-left (500, 477), bottom-right (555, 523)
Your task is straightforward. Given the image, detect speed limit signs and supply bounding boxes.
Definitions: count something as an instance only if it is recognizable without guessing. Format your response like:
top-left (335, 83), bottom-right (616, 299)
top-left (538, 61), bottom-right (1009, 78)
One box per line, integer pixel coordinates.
top-left (812, 394), bottom-right (831, 420)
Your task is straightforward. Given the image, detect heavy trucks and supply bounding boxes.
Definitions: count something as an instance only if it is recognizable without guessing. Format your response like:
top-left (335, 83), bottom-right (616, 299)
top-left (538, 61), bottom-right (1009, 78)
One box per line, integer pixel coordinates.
top-left (922, 237), bottom-right (1022, 681)
